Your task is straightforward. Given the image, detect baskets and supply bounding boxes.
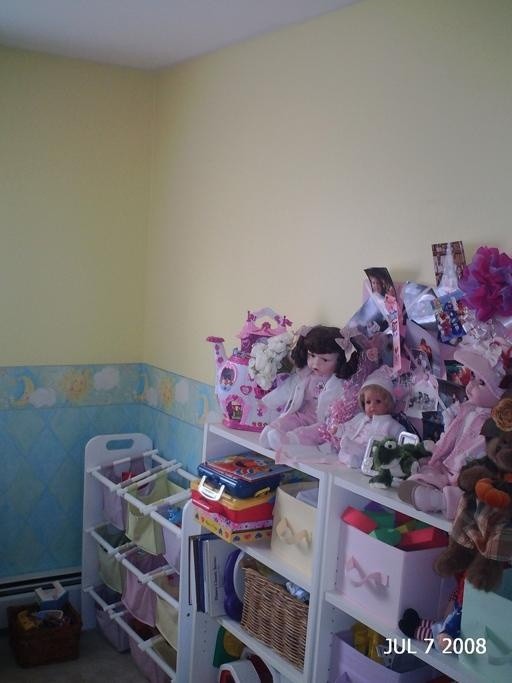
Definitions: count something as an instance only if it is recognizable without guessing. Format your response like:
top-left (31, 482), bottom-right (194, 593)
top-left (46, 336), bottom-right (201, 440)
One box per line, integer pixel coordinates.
top-left (239, 557), bottom-right (310, 674)
top-left (6, 600), bottom-right (84, 669)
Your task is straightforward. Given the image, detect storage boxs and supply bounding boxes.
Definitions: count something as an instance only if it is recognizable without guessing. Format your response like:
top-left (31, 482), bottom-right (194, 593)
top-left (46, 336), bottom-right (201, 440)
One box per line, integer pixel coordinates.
top-left (327, 630), bottom-right (445, 683)
top-left (456, 567), bottom-right (512, 683)
top-left (335, 517), bottom-right (457, 632)
top-left (270, 480), bottom-right (330, 581)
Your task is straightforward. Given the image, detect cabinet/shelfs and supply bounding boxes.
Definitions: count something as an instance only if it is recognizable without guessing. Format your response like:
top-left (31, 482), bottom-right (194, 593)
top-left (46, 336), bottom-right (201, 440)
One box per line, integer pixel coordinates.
top-left (189, 421), bottom-right (512, 683)
